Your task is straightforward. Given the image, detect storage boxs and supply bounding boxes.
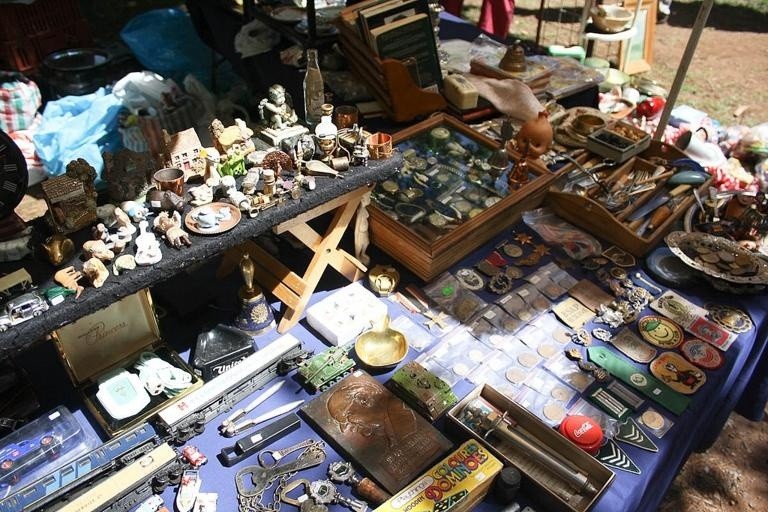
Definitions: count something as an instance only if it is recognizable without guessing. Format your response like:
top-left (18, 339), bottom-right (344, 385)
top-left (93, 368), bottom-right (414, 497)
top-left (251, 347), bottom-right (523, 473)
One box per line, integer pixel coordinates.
top-left (48, 290), bottom-right (163, 388)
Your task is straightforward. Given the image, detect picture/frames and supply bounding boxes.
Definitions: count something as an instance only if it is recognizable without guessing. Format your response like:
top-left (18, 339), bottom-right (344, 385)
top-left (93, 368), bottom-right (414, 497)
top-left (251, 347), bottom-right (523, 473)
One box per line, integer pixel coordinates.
top-left (621, 0), bottom-right (657, 73)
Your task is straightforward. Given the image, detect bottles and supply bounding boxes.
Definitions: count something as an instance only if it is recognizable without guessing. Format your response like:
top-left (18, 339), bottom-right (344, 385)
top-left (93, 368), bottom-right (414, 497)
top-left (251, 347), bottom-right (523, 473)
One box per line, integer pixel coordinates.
top-left (304, 48), bottom-right (325, 125)
top-left (315, 104), bottom-right (338, 161)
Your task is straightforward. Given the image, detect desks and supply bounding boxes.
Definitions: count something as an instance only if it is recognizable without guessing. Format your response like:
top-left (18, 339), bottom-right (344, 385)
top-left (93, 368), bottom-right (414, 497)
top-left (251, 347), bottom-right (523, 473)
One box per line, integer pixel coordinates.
top-left (0, 106), bottom-right (405, 361)
top-left (0, 35), bottom-right (768, 512)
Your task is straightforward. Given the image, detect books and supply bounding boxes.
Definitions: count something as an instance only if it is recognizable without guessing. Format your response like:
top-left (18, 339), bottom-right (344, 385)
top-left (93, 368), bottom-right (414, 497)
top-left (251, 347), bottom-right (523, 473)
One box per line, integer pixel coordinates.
top-left (339, 1), bottom-right (444, 95)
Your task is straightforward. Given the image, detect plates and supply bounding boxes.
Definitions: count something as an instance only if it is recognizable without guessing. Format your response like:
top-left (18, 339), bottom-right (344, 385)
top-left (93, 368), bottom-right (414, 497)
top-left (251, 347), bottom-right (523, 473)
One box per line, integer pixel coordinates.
top-left (185, 202), bottom-right (241, 235)
top-left (269, 5), bottom-right (345, 38)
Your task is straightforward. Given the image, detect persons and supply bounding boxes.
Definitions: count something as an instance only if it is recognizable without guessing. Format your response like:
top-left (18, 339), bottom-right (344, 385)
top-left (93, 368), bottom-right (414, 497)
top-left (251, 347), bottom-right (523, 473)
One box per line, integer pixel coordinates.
top-left (327, 380), bottom-right (417, 452)
